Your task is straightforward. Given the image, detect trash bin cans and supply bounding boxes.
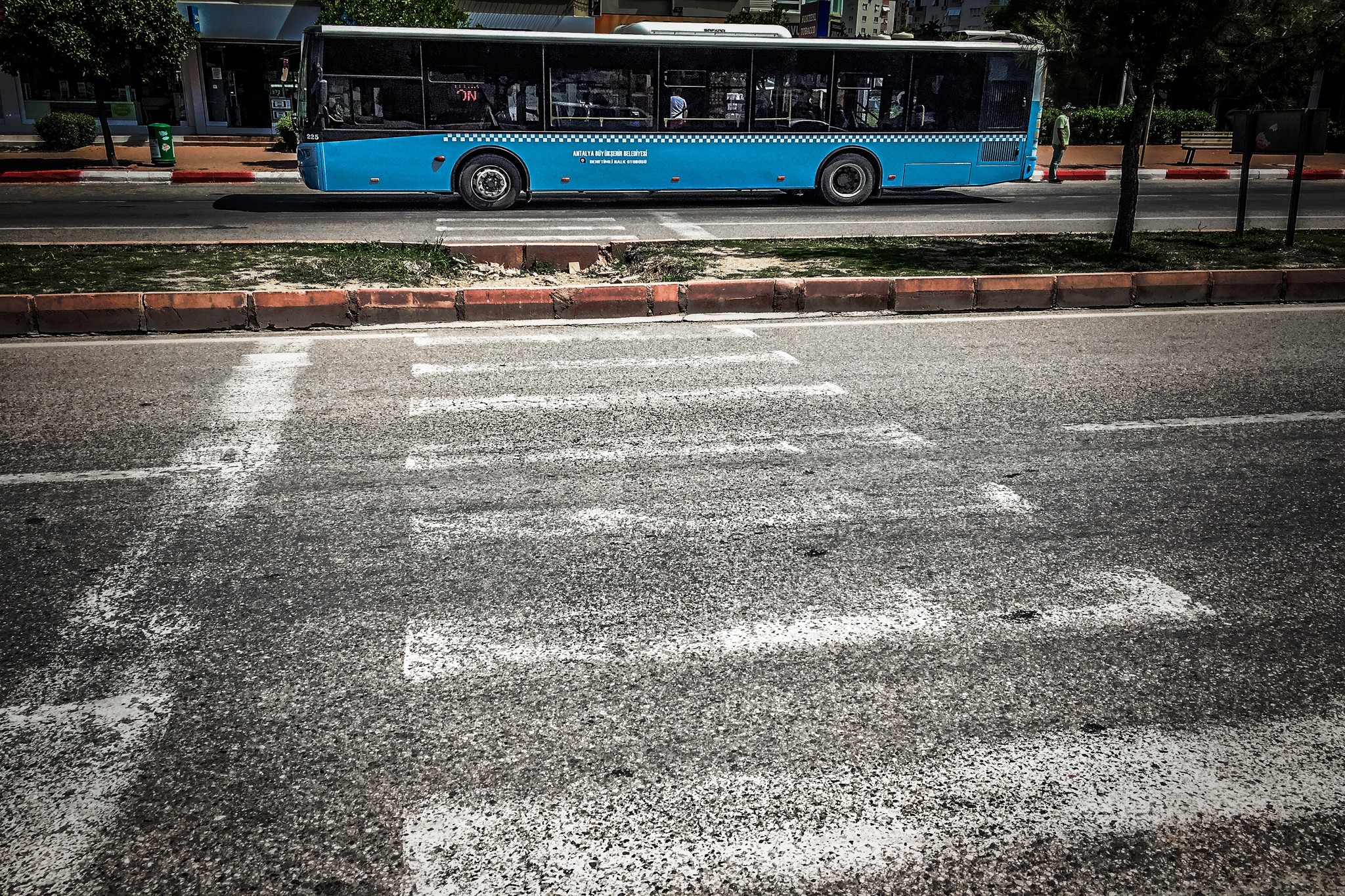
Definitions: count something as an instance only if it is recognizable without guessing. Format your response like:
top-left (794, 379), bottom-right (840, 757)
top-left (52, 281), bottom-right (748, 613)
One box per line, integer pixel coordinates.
top-left (148, 123), bottom-right (176, 165)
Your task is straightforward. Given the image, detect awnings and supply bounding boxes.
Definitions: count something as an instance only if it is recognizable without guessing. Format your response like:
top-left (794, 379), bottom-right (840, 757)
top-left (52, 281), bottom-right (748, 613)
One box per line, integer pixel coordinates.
top-left (882, 7), bottom-right (890, 12)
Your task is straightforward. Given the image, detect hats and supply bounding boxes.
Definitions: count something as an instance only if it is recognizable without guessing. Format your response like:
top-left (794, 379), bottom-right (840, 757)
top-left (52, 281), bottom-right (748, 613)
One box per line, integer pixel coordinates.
top-left (1062, 102), bottom-right (1076, 109)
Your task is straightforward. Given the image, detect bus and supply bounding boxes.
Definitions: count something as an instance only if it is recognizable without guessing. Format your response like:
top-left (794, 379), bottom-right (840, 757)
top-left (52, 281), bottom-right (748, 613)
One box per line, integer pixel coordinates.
top-left (277, 21), bottom-right (1048, 210)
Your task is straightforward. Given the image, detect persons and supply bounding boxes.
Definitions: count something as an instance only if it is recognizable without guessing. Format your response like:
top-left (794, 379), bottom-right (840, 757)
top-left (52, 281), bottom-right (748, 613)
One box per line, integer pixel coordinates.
top-left (1048, 101), bottom-right (1076, 185)
top-left (571, 91), bottom-right (616, 121)
top-left (670, 88), bottom-right (688, 132)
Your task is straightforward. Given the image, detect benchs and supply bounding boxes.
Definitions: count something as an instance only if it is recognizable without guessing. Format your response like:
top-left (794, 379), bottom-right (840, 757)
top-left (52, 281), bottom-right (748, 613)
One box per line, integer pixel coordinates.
top-left (1177, 132), bottom-right (1233, 166)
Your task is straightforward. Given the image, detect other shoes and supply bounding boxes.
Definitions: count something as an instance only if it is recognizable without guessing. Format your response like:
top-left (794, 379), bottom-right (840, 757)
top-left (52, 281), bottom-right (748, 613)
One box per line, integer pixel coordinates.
top-left (1049, 177), bottom-right (1064, 184)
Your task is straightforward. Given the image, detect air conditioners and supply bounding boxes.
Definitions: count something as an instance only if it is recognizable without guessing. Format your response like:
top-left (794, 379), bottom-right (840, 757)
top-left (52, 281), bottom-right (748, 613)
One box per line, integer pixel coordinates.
top-left (958, 0), bottom-right (964, 3)
top-left (932, 15), bottom-right (937, 18)
top-left (941, 6), bottom-right (946, 9)
